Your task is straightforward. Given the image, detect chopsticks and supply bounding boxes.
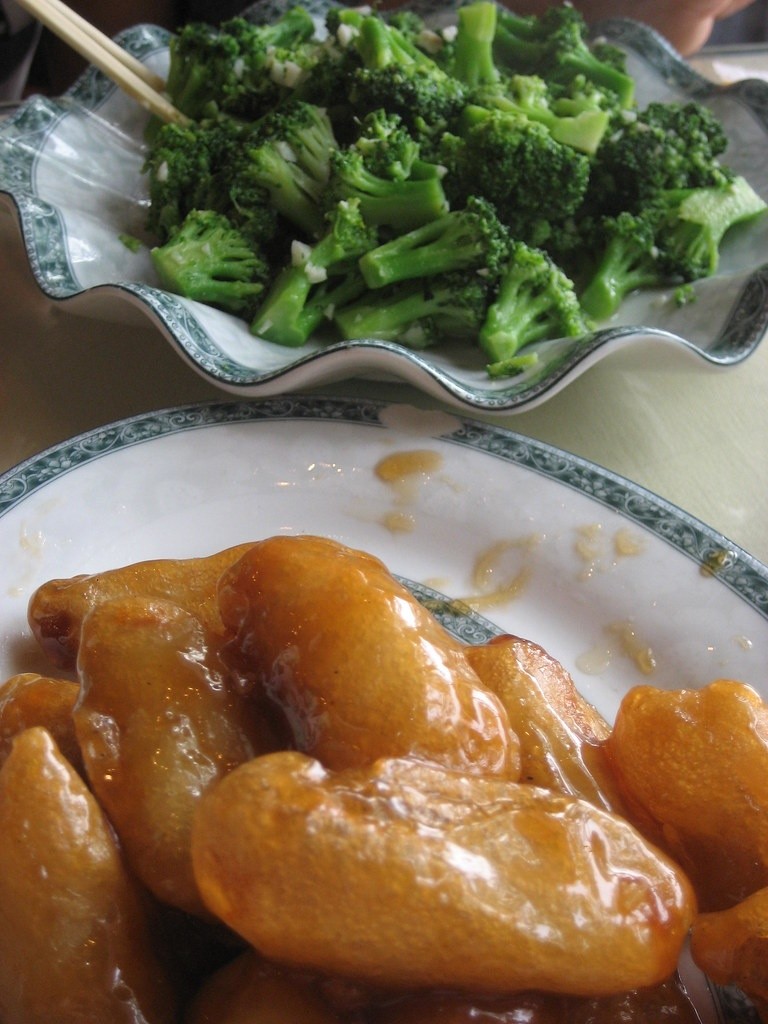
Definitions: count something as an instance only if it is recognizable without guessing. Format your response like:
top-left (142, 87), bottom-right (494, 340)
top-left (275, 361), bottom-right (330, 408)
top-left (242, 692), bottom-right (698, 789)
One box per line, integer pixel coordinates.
top-left (14, 0), bottom-right (194, 131)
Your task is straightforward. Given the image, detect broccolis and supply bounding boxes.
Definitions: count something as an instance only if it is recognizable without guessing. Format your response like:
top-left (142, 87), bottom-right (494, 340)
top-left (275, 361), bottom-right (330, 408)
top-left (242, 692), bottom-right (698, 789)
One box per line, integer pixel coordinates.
top-left (140, 0), bottom-right (768, 379)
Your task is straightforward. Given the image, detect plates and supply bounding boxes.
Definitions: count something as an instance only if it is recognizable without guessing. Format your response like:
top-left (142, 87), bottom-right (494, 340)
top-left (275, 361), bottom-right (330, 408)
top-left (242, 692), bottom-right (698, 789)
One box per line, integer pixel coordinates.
top-left (0, 0), bottom-right (768, 416)
top-left (0, 396), bottom-right (768, 1024)
